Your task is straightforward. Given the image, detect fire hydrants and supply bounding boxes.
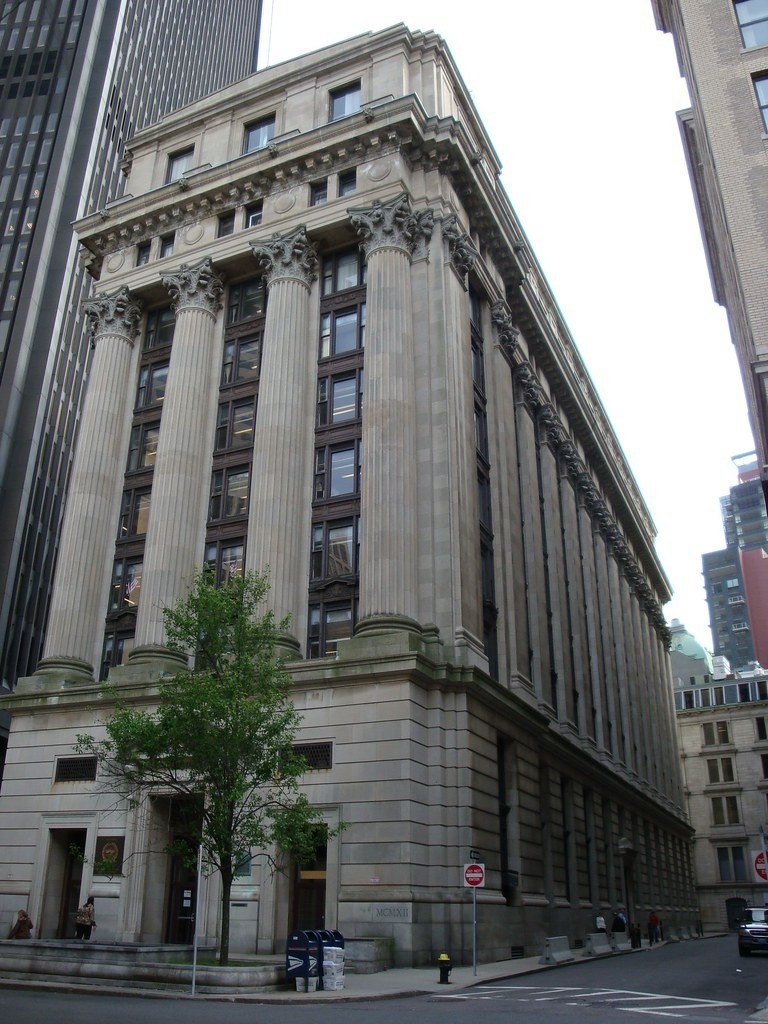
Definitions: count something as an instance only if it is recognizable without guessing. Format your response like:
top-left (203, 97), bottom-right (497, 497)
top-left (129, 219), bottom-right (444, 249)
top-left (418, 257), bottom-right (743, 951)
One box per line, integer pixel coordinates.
top-left (438, 953), bottom-right (452, 984)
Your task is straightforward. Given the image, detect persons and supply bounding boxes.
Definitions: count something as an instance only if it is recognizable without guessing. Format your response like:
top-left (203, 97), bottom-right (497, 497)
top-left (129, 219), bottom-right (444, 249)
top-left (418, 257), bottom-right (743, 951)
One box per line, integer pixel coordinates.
top-left (6, 908), bottom-right (34, 941)
top-left (74, 895), bottom-right (97, 940)
top-left (610, 908), bottom-right (628, 933)
top-left (649, 911), bottom-right (660, 943)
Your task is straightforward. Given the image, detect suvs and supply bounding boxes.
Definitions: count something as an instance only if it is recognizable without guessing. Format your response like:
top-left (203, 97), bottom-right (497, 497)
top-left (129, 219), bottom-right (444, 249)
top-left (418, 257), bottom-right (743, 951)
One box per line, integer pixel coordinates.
top-left (738, 906), bottom-right (768, 958)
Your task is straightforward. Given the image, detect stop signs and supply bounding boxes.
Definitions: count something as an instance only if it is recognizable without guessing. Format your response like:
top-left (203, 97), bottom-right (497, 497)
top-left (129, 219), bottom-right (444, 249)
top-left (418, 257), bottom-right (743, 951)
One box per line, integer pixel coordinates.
top-left (463, 863), bottom-right (484, 887)
top-left (751, 850), bottom-right (768, 883)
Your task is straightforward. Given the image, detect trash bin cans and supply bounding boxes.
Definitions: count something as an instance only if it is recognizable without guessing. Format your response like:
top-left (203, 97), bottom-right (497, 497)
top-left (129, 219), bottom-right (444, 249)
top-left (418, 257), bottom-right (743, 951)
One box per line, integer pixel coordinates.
top-left (285, 927), bottom-right (346, 994)
